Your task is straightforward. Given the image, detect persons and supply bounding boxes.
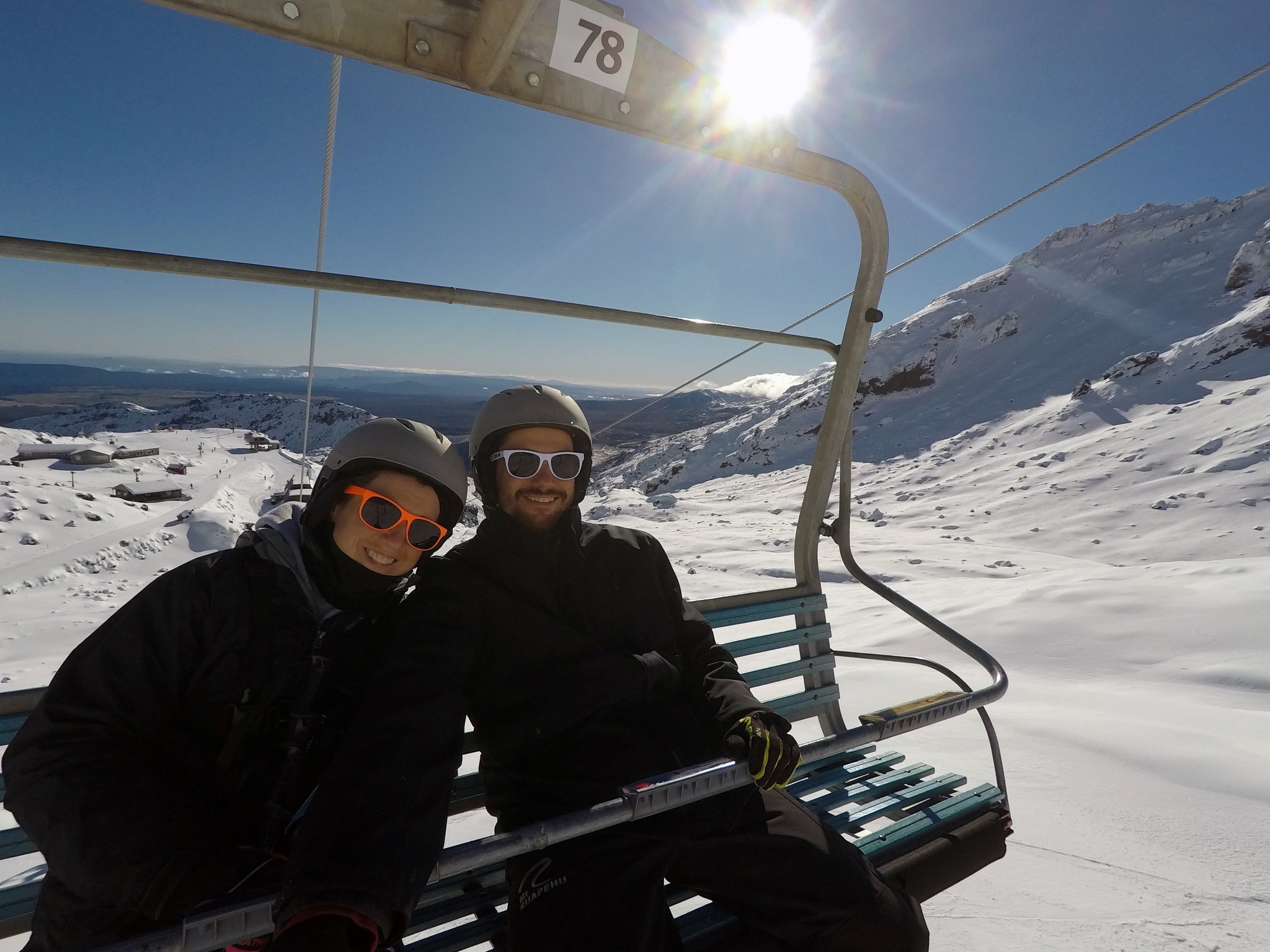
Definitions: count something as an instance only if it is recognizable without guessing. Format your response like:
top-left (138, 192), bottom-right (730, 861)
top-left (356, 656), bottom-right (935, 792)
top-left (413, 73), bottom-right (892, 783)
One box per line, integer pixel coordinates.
top-left (415, 382), bottom-right (929, 952)
top-left (1, 415), bottom-right (468, 952)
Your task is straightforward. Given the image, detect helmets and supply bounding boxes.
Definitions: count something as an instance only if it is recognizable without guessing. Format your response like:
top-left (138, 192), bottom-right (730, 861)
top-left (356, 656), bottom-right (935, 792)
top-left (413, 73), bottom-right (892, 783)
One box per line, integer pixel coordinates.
top-left (467, 383), bottom-right (593, 508)
top-left (310, 416), bottom-right (468, 552)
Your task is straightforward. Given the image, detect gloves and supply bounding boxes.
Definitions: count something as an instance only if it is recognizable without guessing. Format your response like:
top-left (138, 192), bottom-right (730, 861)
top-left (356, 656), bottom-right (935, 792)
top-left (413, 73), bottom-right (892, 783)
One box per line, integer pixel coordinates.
top-left (269, 902), bottom-right (382, 952)
top-left (725, 712), bottom-right (803, 789)
top-left (139, 845), bottom-right (290, 924)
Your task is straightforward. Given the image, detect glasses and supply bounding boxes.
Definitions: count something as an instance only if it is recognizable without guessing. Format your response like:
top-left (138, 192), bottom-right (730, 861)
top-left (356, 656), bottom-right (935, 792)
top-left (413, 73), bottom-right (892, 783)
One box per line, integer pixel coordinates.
top-left (490, 449), bottom-right (585, 481)
top-left (342, 481), bottom-right (448, 551)
